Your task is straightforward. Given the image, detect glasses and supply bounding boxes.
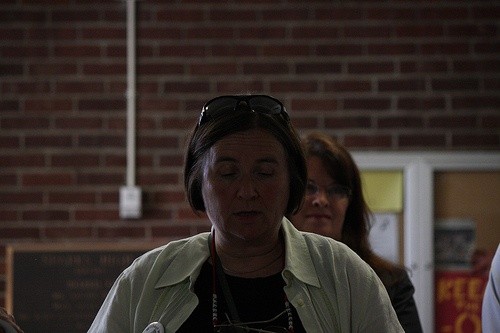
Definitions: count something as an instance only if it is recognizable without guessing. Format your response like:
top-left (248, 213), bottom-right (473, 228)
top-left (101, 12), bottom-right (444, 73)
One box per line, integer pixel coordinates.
top-left (198, 93), bottom-right (290, 128)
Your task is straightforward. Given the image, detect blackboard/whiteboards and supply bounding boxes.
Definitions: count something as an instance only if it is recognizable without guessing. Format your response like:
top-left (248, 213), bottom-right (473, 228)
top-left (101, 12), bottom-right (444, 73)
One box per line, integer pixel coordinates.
top-left (3, 238), bottom-right (168, 333)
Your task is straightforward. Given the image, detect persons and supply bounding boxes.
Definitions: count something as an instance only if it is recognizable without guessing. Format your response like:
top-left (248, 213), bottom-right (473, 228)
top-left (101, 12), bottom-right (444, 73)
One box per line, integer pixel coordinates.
top-left (283, 132), bottom-right (424, 332)
top-left (89, 93), bottom-right (407, 333)
